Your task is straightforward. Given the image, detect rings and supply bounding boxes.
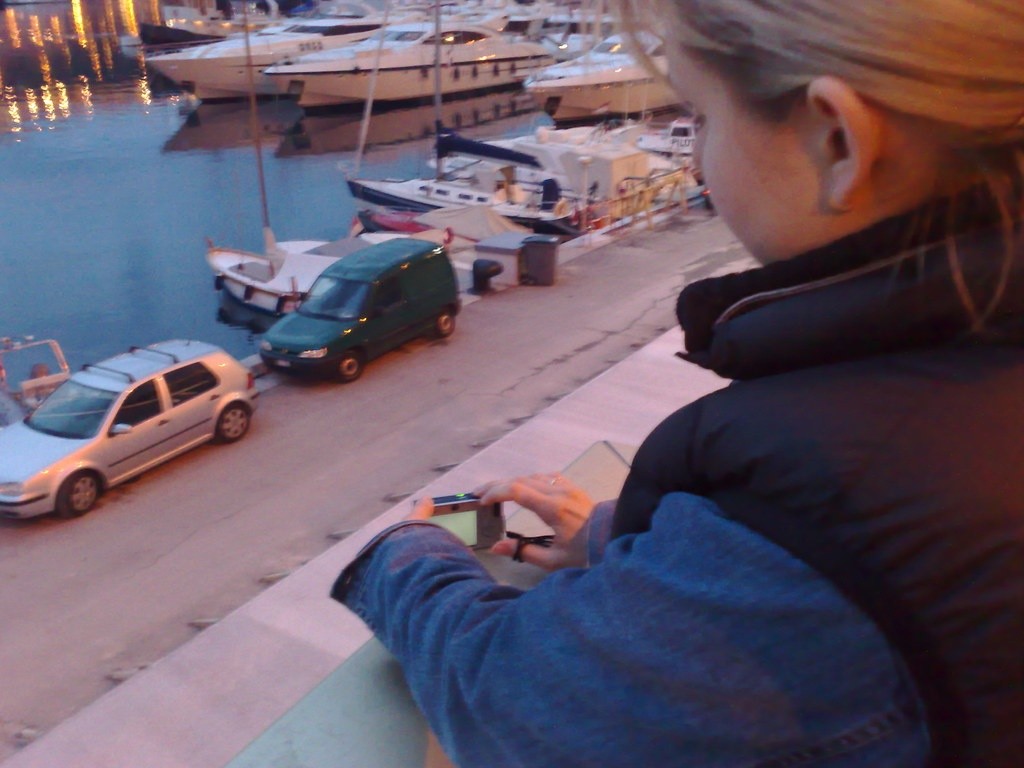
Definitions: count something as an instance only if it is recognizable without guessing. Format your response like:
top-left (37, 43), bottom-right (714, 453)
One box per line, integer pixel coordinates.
top-left (550, 478), bottom-right (562, 485)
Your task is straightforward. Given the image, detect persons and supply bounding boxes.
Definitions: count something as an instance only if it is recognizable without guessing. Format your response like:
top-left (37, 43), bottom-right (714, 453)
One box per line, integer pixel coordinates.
top-left (603, 118), bottom-right (622, 130)
top-left (328, 0), bottom-right (1024, 768)
top-left (21, 363), bottom-right (56, 409)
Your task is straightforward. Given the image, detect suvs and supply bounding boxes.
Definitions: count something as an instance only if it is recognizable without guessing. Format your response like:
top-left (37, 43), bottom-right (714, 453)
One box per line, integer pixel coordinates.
top-left (260, 238), bottom-right (462, 383)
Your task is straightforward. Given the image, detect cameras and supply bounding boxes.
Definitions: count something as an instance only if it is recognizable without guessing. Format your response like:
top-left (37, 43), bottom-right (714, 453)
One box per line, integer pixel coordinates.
top-left (412, 492), bottom-right (506, 551)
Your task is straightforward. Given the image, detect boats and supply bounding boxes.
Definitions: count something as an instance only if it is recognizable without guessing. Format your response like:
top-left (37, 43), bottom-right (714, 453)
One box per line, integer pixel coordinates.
top-left (200, 0), bottom-right (341, 315)
top-left (138, 0), bottom-right (695, 291)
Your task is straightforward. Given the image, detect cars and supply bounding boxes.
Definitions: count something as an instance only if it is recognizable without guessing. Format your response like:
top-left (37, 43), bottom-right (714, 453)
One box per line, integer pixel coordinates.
top-left (0, 339), bottom-right (260, 520)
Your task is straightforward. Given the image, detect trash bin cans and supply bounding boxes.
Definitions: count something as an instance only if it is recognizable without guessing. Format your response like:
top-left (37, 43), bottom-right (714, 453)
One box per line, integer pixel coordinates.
top-left (521, 234), bottom-right (559, 285)
